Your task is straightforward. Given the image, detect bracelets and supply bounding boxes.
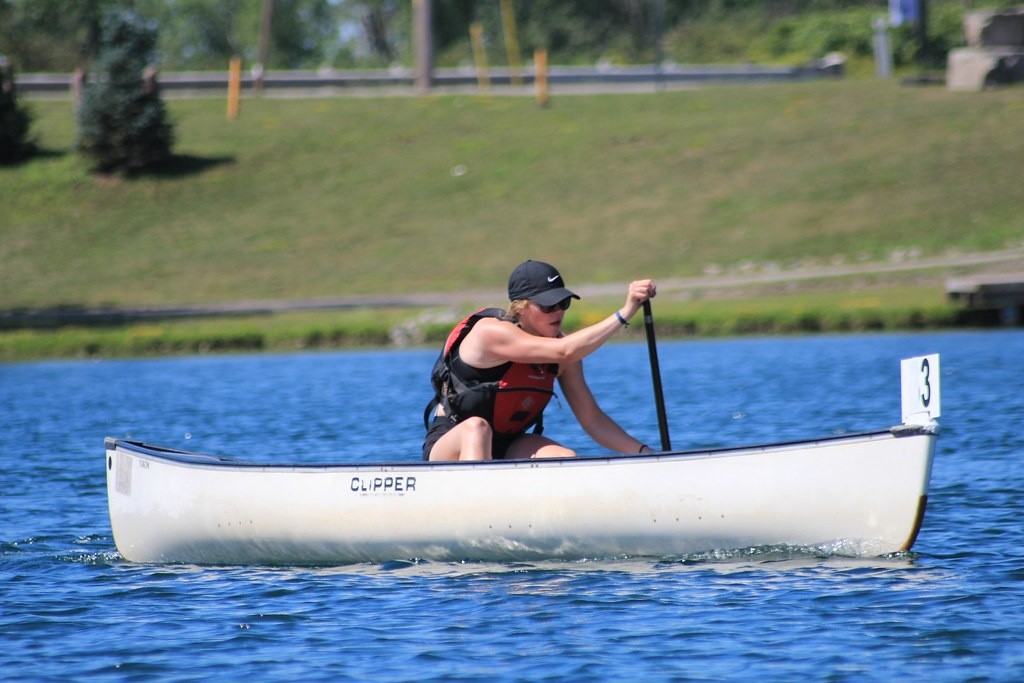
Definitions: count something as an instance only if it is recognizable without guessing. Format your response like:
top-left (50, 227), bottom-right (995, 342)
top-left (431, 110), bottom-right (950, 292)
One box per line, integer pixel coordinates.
top-left (616, 310), bottom-right (630, 328)
top-left (639, 445), bottom-right (647, 453)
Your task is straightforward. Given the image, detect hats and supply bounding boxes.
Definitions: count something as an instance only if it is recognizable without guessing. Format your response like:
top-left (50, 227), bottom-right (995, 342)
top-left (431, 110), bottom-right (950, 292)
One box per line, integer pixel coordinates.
top-left (508, 260), bottom-right (580, 307)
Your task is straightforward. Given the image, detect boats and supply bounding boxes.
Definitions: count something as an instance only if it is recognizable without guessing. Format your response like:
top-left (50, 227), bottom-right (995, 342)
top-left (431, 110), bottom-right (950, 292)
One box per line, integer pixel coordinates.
top-left (106, 413), bottom-right (942, 569)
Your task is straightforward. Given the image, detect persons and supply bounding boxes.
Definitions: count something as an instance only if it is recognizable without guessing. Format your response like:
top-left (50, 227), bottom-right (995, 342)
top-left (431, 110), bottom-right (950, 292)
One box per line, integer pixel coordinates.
top-left (422, 259), bottom-right (657, 462)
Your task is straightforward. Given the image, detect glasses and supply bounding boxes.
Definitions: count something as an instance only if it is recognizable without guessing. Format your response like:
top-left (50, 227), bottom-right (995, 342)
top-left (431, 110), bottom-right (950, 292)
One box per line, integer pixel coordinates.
top-left (540, 297), bottom-right (571, 314)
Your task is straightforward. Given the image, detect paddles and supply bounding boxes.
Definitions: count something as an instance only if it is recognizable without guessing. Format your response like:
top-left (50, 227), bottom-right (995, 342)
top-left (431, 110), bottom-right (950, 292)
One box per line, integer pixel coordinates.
top-left (637, 291), bottom-right (679, 450)
